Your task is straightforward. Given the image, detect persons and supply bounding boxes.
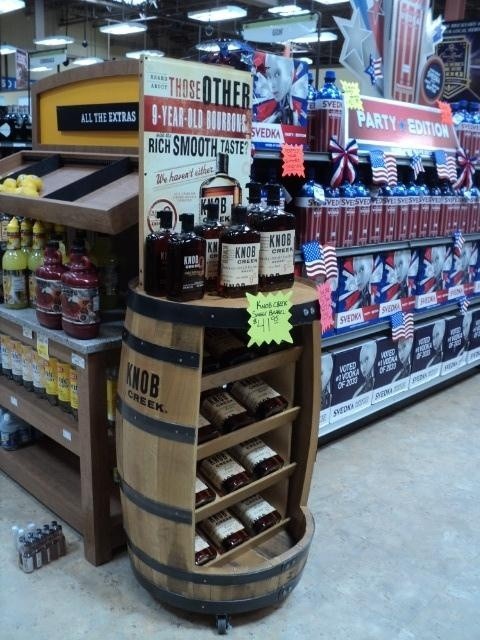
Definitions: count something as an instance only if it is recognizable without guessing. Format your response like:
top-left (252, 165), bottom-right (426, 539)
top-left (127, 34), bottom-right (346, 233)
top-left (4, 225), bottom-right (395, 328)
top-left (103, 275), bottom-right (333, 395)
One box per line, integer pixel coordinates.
top-left (301, 241), bottom-right (480, 409)
top-left (260, 53), bottom-right (300, 126)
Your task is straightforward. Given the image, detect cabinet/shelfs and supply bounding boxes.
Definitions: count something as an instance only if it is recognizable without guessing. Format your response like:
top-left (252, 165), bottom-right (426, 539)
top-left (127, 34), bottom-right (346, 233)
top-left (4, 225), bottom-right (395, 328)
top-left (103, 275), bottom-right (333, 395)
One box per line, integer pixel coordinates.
top-left (0, 56), bottom-right (139, 568)
top-left (253, 145), bottom-right (480, 449)
top-left (115, 278), bottom-right (321, 634)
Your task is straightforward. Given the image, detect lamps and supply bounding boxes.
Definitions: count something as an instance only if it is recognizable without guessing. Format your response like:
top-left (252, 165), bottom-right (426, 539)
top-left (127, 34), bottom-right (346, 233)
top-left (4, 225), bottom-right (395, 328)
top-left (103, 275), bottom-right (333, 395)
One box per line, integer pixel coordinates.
top-left (290, 32), bottom-right (338, 43)
top-left (33, 35), bottom-right (74, 46)
top-left (268, 0), bottom-right (314, 16)
top-left (188, 5), bottom-right (247, 23)
top-left (195, 38), bottom-right (250, 53)
top-left (100, 22), bottom-right (148, 35)
top-left (1, 45), bottom-right (15, 55)
top-left (0, 0), bottom-right (27, 15)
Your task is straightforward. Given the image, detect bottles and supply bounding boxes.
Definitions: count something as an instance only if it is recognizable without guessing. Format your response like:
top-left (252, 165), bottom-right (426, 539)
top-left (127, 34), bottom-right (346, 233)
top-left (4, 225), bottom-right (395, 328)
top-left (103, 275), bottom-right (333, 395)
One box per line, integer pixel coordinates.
top-left (198, 203), bottom-right (226, 293)
top-left (198, 153), bottom-right (242, 228)
top-left (169, 214), bottom-right (208, 302)
top-left (0, 333), bottom-right (118, 453)
top-left (448, 97), bottom-right (480, 163)
top-left (16, 519), bottom-right (69, 574)
top-left (242, 181), bottom-right (265, 228)
top-left (298, 168), bottom-right (479, 253)
top-left (305, 68), bottom-right (342, 152)
top-left (142, 209), bottom-right (176, 297)
top-left (217, 205), bottom-right (261, 299)
top-left (258, 184), bottom-right (298, 292)
top-left (195, 331), bottom-right (305, 564)
top-left (0, 212), bottom-right (132, 341)
top-left (6, 104), bottom-right (33, 144)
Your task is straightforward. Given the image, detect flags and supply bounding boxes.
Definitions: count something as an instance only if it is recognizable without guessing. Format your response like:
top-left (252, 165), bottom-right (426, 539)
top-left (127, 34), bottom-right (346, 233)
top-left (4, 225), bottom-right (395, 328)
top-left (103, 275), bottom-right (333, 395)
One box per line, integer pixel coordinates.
top-left (434, 150), bottom-right (457, 183)
top-left (411, 156), bottom-right (425, 181)
top-left (369, 149), bottom-right (398, 186)
